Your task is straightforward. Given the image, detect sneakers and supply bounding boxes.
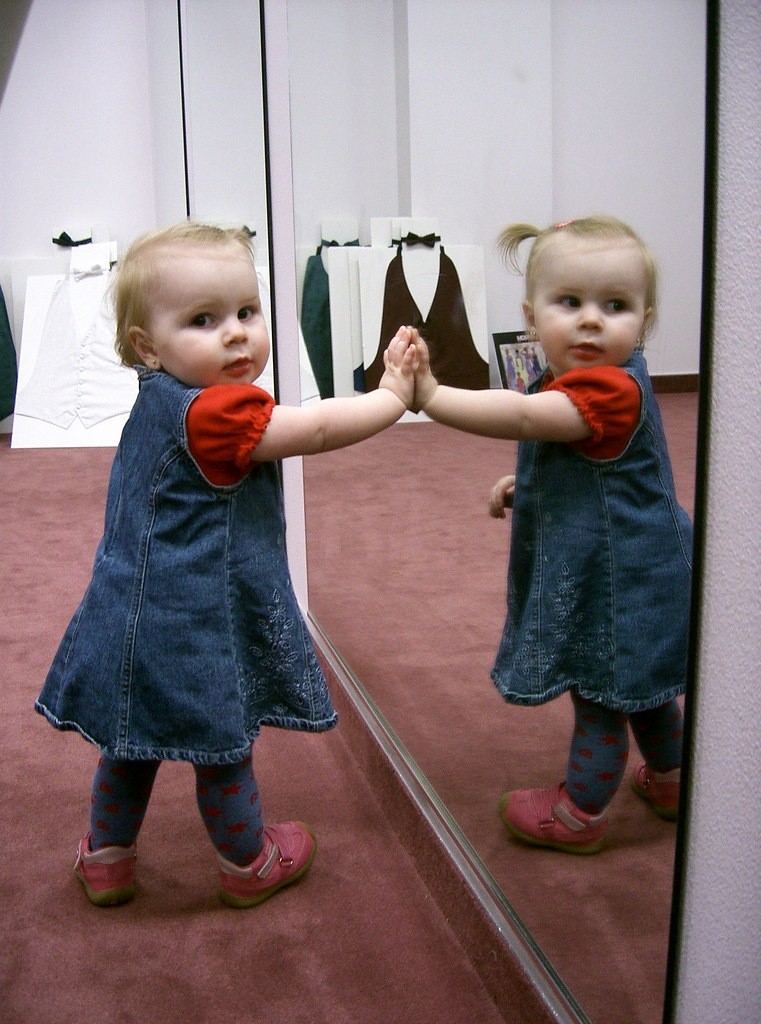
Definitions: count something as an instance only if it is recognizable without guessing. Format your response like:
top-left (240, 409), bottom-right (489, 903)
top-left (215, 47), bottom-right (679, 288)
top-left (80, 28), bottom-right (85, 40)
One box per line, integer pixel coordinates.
top-left (628, 765), bottom-right (683, 819)
top-left (497, 784), bottom-right (605, 856)
top-left (73, 833), bottom-right (138, 908)
top-left (215, 821), bottom-right (317, 908)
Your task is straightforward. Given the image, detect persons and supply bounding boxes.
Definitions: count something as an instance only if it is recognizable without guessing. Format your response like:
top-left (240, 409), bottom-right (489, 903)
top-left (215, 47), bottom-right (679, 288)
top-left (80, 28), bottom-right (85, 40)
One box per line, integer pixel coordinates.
top-left (407, 210), bottom-right (697, 858)
top-left (30, 219), bottom-right (421, 908)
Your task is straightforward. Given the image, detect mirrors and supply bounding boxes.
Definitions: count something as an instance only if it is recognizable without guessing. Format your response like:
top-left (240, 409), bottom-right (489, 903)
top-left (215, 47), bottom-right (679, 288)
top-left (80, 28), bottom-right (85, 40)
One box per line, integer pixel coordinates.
top-left (255, 0), bottom-right (718, 1023)
top-left (175, 1), bottom-right (278, 416)
